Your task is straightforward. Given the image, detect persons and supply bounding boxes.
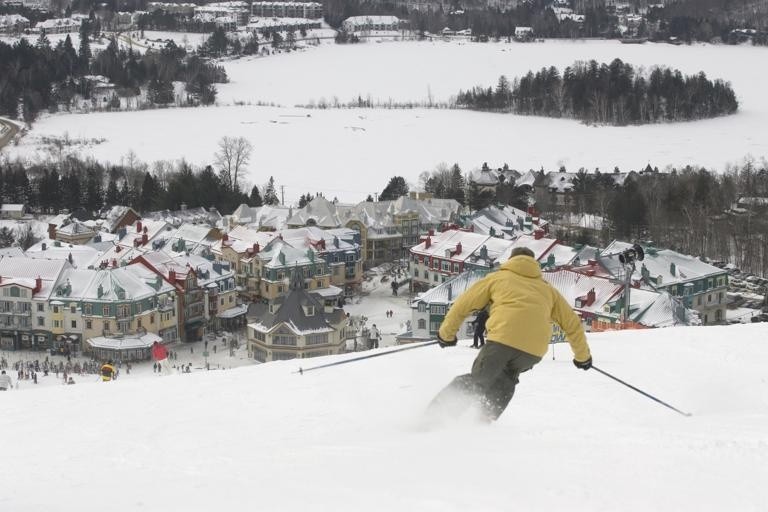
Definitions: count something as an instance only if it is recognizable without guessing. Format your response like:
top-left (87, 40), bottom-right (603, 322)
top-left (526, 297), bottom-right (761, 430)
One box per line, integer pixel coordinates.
top-left (154, 363), bottom-right (157, 372)
top-left (0, 356), bottom-right (117, 391)
top-left (470, 307), bottom-right (489, 348)
top-left (205, 340), bottom-right (208, 349)
top-left (386, 310), bottom-right (389, 318)
top-left (120, 360), bottom-right (122, 368)
top-left (429, 248), bottom-right (592, 422)
top-left (389, 310), bottom-right (393, 317)
top-left (158, 363), bottom-right (161, 372)
top-left (126, 368), bottom-right (130, 374)
top-left (369, 324), bottom-right (382, 349)
top-left (166, 347), bottom-right (193, 372)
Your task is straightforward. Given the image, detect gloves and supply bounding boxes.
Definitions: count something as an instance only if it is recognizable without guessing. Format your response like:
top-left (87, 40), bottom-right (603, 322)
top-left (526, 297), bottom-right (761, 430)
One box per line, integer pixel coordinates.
top-left (573, 357), bottom-right (593, 370)
top-left (436, 331), bottom-right (458, 349)
